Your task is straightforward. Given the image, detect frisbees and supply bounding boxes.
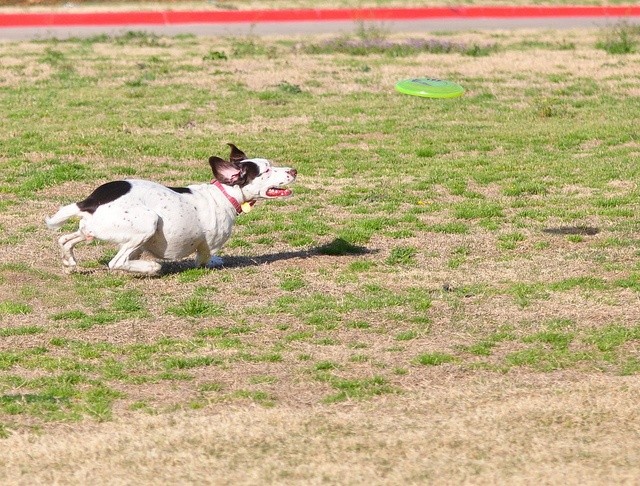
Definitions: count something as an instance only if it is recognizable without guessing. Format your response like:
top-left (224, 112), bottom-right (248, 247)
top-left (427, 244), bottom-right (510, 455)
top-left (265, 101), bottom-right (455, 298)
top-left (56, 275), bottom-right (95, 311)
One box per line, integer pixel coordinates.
top-left (394, 78), bottom-right (465, 99)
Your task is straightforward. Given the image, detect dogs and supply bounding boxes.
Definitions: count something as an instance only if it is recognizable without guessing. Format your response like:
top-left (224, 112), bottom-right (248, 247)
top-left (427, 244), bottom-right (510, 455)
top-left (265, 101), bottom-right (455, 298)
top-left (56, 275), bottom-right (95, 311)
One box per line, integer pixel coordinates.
top-left (43, 143), bottom-right (297, 277)
